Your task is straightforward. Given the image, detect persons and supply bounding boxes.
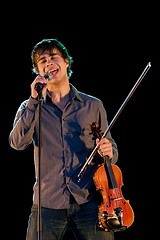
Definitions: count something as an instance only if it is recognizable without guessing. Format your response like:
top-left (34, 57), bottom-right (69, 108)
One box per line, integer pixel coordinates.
top-left (7, 38), bottom-right (120, 239)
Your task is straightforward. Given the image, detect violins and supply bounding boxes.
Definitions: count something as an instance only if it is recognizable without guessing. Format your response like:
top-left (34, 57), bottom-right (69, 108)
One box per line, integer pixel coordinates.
top-left (90, 122), bottom-right (135, 232)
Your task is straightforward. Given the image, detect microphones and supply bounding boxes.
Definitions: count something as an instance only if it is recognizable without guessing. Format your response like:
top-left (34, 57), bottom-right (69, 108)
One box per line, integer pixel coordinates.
top-left (35, 73), bottom-right (53, 91)
